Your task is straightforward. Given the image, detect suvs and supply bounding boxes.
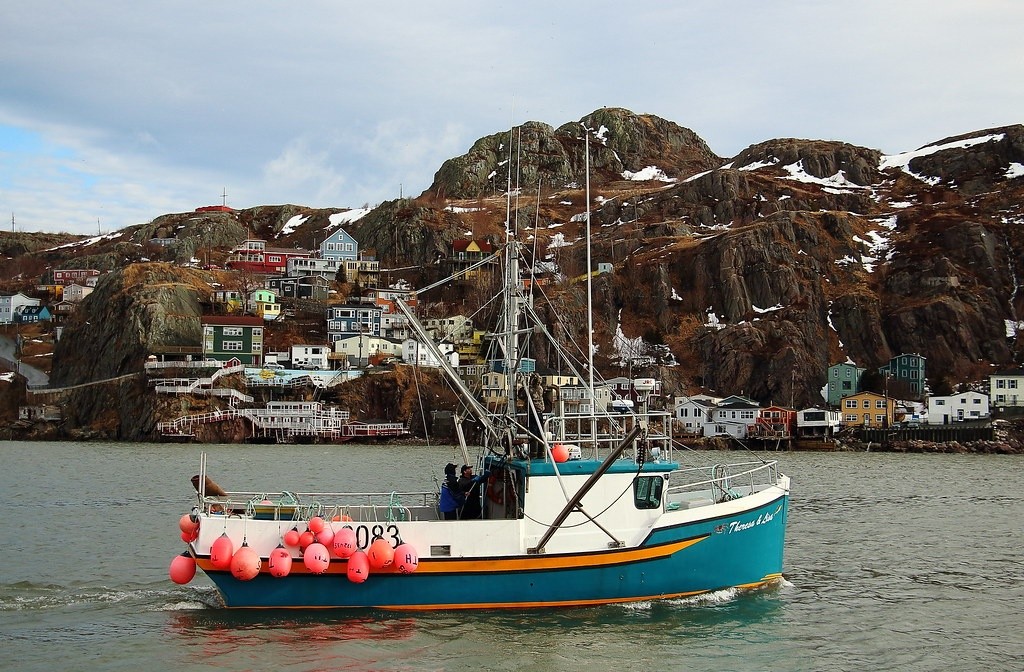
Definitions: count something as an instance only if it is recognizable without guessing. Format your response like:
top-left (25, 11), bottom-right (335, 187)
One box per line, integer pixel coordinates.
top-left (382, 357), bottom-right (402, 364)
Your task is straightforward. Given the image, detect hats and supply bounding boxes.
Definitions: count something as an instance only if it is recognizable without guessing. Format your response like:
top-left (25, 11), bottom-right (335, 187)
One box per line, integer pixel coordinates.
top-left (461, 465), bottom-right (473, 474)
top-left (445, 463), bottom-right (457, 475)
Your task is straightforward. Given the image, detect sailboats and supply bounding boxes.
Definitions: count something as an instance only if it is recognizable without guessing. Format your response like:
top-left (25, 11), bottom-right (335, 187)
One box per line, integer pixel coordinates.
top-left (189, 118), bottom-right (790, 609)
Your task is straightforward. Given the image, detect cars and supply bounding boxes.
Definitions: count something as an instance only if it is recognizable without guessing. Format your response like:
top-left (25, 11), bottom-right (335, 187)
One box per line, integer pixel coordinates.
top-left (263, 362), bottom-right (285, 370)
top-left (907, 420), bottom-right (918, 426)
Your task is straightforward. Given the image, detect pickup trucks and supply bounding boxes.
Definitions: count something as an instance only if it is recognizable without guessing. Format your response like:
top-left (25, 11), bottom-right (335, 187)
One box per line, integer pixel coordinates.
top-left (295, 360), bottom-right (321, 371)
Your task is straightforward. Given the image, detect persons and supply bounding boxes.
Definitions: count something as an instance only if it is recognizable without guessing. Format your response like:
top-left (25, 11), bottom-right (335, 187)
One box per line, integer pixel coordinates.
top-left (458, 464), bottom-right (490, 519)
top-left (518, 373), bottom-right (550, 458)
top-left (439, 462), bottom-right (464, 520)
